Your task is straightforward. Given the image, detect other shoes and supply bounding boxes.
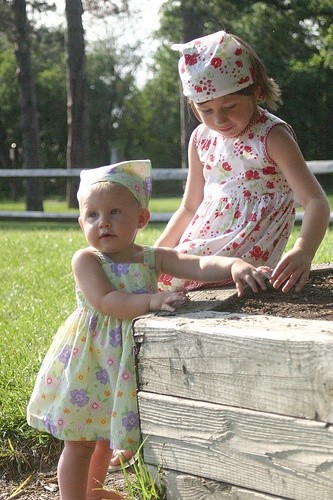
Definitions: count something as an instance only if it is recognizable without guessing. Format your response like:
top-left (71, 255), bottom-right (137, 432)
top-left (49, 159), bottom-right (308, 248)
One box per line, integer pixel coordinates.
top-left (108, 449), bottom-right (142, 471)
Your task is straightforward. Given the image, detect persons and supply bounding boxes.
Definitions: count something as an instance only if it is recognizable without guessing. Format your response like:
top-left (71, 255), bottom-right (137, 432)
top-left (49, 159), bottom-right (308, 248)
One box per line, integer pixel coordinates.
top-left (148, 30), bottom-right (331, 294)
top-left (24, 158), bottom-right (274, 500)
